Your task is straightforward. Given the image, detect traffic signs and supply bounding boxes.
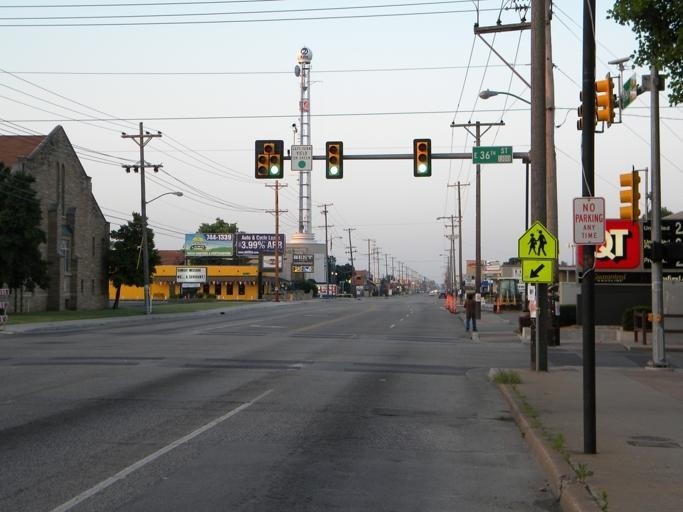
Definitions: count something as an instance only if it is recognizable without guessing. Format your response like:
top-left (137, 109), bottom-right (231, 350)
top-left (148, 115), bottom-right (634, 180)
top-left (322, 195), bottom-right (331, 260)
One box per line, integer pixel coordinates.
top-left (290, 144), bottom-right (311, 171)
top-left (519, 221), bottom-right (557, 282)
top-left (573, 196), bottom-right (606, 246)
top-left (473, 146), bottom-right (512, 165)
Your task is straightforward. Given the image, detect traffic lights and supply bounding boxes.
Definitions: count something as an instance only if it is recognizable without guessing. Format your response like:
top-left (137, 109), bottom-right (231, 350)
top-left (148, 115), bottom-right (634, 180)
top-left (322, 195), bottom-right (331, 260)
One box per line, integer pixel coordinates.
top-left (591, 71), bottom-right (615, 126)
top-left (621, 73), bottom-right (636, 110)
top-left (269, 154), bottom-right (280, 177)
top-left (575, 90), bottom-right (582, 130)
top-left (414, 139), bottom-right (432, 178)
top-left (618, 165), bottom-right (641, 223)
top-left (263, 143), bottom-right (274, 154)
top-left (257, 154), bottom-right (269, 176)
top-left (326, 141), bottom-right (343, 178)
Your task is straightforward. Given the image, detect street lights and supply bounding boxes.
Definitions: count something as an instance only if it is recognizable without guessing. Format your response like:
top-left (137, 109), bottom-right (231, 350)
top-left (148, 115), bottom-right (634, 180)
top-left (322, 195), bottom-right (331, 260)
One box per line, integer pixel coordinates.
top-left (331, 272), bottom-right (337, 298)
top-left (325, 236), bottom-right (343, 254)
top-left (439, 253), bottom-right (449, 290)
top-left (477, 87), bottom-right (531, 109)
top-left (145, 190), bottom-right (183, 204)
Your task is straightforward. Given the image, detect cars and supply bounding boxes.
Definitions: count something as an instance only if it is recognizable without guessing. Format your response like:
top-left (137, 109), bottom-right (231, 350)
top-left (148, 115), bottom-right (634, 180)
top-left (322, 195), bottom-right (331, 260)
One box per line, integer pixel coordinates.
top-left (428, 289), bottom-right (447, 299)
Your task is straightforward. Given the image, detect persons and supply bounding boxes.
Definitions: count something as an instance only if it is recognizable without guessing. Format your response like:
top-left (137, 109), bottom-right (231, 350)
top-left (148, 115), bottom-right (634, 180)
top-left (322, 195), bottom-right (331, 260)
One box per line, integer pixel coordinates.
top-left (463, 292), bottom-right (478, 332)
top-left (534, 229), bottom-right (548, 256)
top-left (526, 232), bottom-right (537, 255)
